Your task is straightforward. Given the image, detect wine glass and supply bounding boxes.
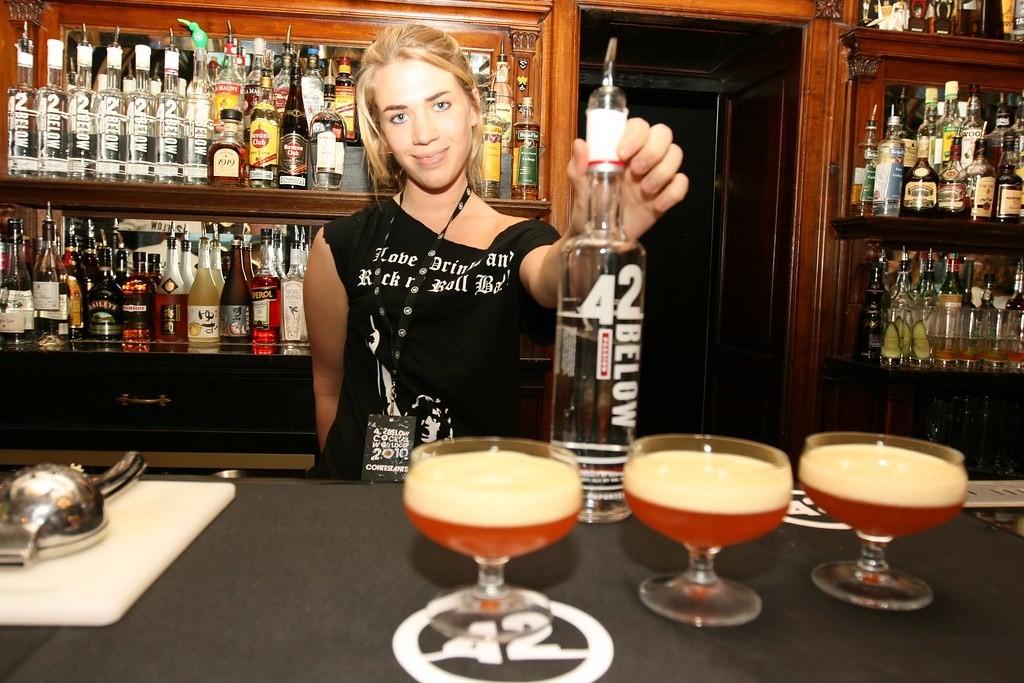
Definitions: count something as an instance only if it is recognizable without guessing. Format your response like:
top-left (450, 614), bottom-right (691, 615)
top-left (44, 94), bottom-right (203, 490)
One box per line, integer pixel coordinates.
top-left (795, 431), bottom-right (967, 610)
top-left (401, 435), bottom-right (583, 639)
top-left (619, 434), bottom-right (791, 626)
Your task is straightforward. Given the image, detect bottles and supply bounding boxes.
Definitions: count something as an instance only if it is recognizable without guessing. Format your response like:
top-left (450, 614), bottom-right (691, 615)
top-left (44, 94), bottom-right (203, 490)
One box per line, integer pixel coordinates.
top-left (841, 0), bottom-right (1024, 370)
top-left (546, 79), bottom-right (645, 524)
top-left (0, 32), bottom-right (542, 351)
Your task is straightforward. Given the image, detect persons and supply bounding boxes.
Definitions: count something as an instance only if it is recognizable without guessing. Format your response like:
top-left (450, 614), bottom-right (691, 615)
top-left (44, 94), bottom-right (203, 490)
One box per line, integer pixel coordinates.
top-left (303, 24), bottom-right (689, 481)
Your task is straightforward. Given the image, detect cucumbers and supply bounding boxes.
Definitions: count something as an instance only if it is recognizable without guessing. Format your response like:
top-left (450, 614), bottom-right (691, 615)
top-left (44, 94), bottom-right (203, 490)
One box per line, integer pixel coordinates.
top-left (880, 317), bottom-right (931, 360)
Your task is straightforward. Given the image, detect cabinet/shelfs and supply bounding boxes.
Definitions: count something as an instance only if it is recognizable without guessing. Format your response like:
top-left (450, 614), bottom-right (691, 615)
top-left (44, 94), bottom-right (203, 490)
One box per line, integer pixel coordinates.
top-left (821, 27), bottom-right (1024, 475)
top-left (0, 0), bottom-right (580, 475)
